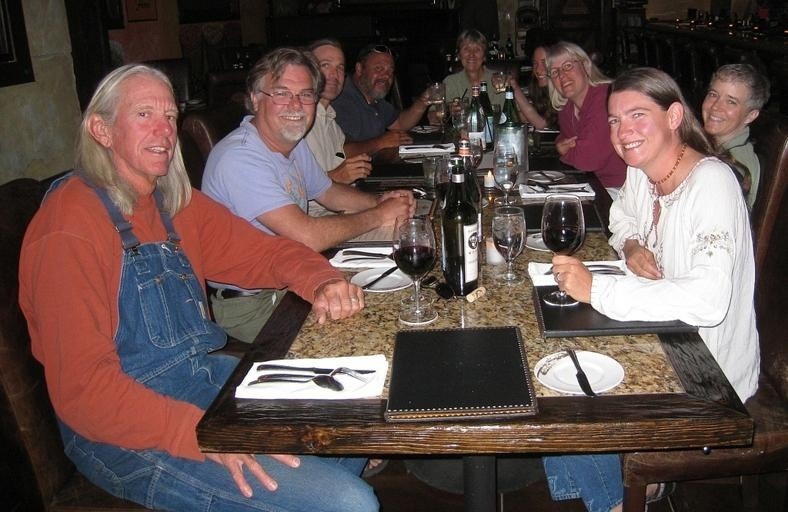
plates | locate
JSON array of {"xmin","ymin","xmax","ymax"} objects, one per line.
[
  {"xmin": 528, "ymin": 169, "xmax": 566, "ymax": 183},
  {"xmin": 523, "ymin": 231, "xmax": 557, "ymax": 254},
  {"xmin": 349, "ymin": 267, "xmax": 418, "ymax": 295},
  {"xmin": 531, "ymin": 348, "xmax": 627, "ymax": 396},
  {"xmin": 411, "ymin": 125, "xmax": 436, "ymax": 134}
]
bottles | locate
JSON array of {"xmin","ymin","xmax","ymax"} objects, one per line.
[
  {"xmin": 440, "ymin": 140, "xmax": 479, "ymax": 295},
  {"xmin": 465, "ymin": 82, "xmax": 521, "ymax": 153}
]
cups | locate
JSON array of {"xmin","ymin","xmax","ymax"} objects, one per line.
[
  {"xmin": 422, "ymin": 153, "xmax": 443, "ymax": 190},
  {"xmin": 449, "ymin": 97, "xmax": 470, "ymax": 130},
  {"xmin": 493, "ymin": 120, "xmax": 530, "ymax": 170},
  {"xmin": 424, "ymin": 71, "xmax": 511, "ymax": 105}
]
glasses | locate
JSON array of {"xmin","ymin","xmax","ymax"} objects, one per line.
[
  {"xmin": 549, "ymin": 60, "xmax": 580, "ymax": 80},
  {"xmin": 259, "ymin": 90, "xmax": 318, "ymax": 105},
  {"xmin": 372, "ymin": 45, "xmax": 392, "ymax": 55}
]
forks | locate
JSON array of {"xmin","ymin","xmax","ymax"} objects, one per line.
[
  {"xmin": 258, "ymin": 367, "xmax": 368, "ymax": 383},
  {"xmin": 405, "ymin": 143, "xmax": 447, "ymax": 150}
]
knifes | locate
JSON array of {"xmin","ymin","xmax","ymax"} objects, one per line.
[
  {"xmin": 257, "ymin": 362, "xmax": 377, "ymax": 374},
  {"xmin": 564, "ymin": 345, "xmax": 596, "ymax": 397},
  {"xmin": 360, "ymin": 267, "xmax": 399, "ymax": 289}
]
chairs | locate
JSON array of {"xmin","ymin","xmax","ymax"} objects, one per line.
[
  {"xmin": 0, "ymin": 166, "xmax": 164, "ymax": 511},
  {"xmin": 180, "ymin": 97, "xmax": 243, "ymax": 154},
  {"xmin": 619, "ymin": 310, "xmax": 786, "ymax": 511},
  {"xmin": 742, "ymin": 118, "xmax": 786, "ymax": 287}
]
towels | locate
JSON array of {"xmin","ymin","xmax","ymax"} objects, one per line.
[
  {"xmin": 328, "ymin": 246, "xmax": 398, "ymax": 270},
  {"xmin": 230, "ymin": 351, "xmax": 389, "ymax": 399},
  {"xmin": 517, "ymin": 182, "xmax": 597, "ymax": 201},
  {"xmin": 526, "ymin": 258, "xmax": 634, "ymax": 288},
  {"xmin": 395, "ymin": 141, "xmax": 458, "ymax": 156}
]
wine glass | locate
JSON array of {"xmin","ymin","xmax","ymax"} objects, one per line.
[
  {"xmin": 493, "ymin": 146, "xmax": 518, "ymax": 204},
  {"xmin": 434, "ymin": 104, "xmax": 451, "ymax": 138},
  {"xmin": 392, "ymin": 211, "xmax": 439, "ymax": 326},
  {"xmin": 490, "ymin": 206, "xmax": 528, "ymax": 286},
  {"xmin": 540, "ymin": 194, "xmax": 587, "ymax": 307}
]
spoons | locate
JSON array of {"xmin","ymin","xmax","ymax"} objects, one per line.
[
  {"xmin": 246, "ymin": 375, "xmax": 344, "ymax": 393},
  {"xmin": 342, "ymin": 249, "xmax": 395, "ymax": 260}
]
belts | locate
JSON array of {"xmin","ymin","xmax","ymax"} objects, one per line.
[{"xmin": 206, "ymin": 285, "xmax": 260, "ymax": 299}]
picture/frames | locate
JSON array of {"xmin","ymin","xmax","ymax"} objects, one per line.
[
  {"xmin": 124, "ymin": 0, "xmax": 159, "ymax": 26},
  {"xmin": 0, "ymin": 0, "xmax": 35, "ymax": 90}
]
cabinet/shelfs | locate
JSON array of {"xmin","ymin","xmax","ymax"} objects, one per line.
[{"xmin": 63, "ymin": 1, "xmax": 242, "ymax": 180}]
